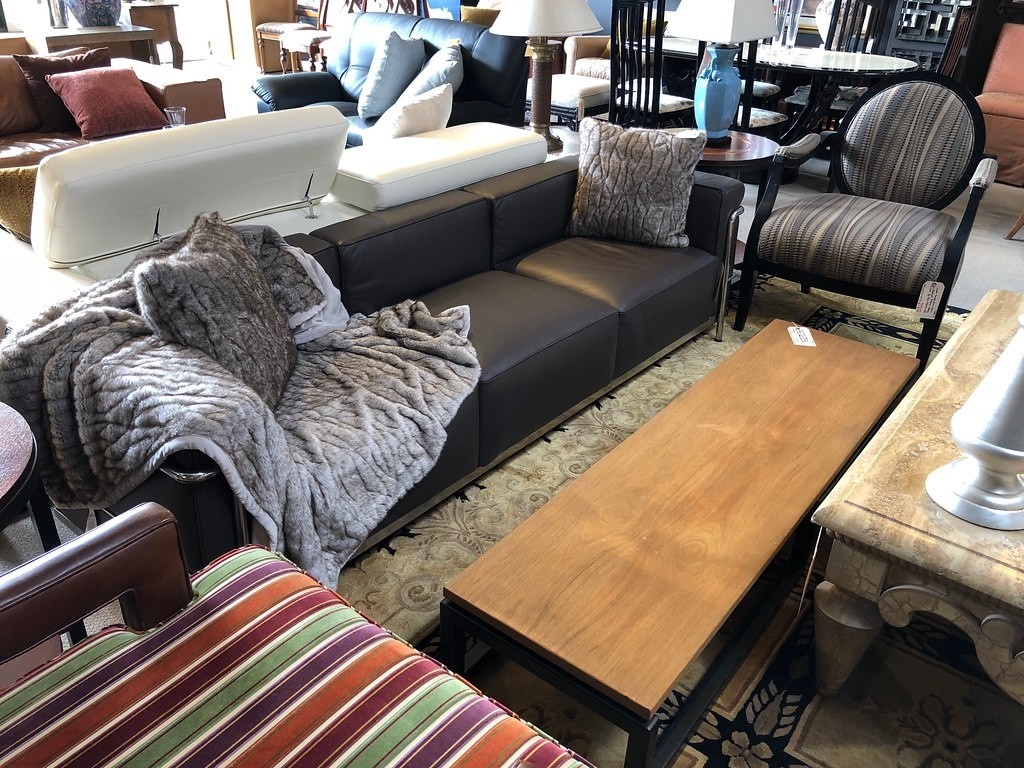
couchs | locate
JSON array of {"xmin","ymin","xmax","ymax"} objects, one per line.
[
  {"xmin": 0, "ymin": 104, "xmax": 545, "ymax": 335},
  {"xmin": 251, "ymin": 16, "xmax": 531, "ymax": 149},
  {"xmin": 56, "ymin": 151, "xmax": 745, "ymax": 551},
  {"xmin": 1, "ymin": 47, "xmax": 226, "ymax": 169}
]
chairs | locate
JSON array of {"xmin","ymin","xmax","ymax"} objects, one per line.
[
  {"xmin": 256, "ymin": 0, "xmax": 414, "ymax": 74},
  {"xmin": 0, "ymin": 499, "xmax": 600, "ymax": 768},
  {"xmin": 608, "ymin": 1, "xmax": 1004, "ymax": 352}
]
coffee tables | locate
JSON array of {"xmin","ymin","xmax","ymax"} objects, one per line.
[{"xmin": 439, "ymin": 319, "xmax": 920, "ymax": 768}]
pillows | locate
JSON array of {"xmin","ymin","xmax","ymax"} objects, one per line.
[
  {"xmin": 12, "ymin": 46, "xmax": 170, "ymax": 140},
  {"xmin": 573, "ymin": 109, "xmax": 706, "ymax": 251},
  {"xmin": 355, "ymin": 30, "xmax": 465, "ymax": 145},
  {"xmin": 132, "ymin": 212, "xmax": 299, "ymax": 419}
]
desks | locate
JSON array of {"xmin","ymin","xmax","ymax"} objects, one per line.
[
  {"xmin": 811, "ymin": 289, "xmax": 1024, "ymax": 698},
  {"xmin": 44, "ymin": 4, "xmax": 185, "ymax": 69},
  {"xmin": 625, "ymin": 35, "xmax": 917, "ymax": 177}
]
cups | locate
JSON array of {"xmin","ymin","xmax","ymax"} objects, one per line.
[
  {"xmin": 164, "ymin": 107, "xmax": 187, "ymax": 124},
  {"xmin": 162, "ymin": 124, "xmax": 185, "ymax": 129}
]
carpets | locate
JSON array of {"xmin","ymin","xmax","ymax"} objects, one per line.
[{"xmin": 327, "ymin": 303, "xmax": 1024, "ymax": 768}]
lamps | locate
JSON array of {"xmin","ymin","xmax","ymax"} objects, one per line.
[
  {"xmin": 665, "ymin": 1, "xmax": 779, "ymax": 148},
  {"xmin": 488, "ymin": 0, "xmax": 604, "ymax": 152}
]
